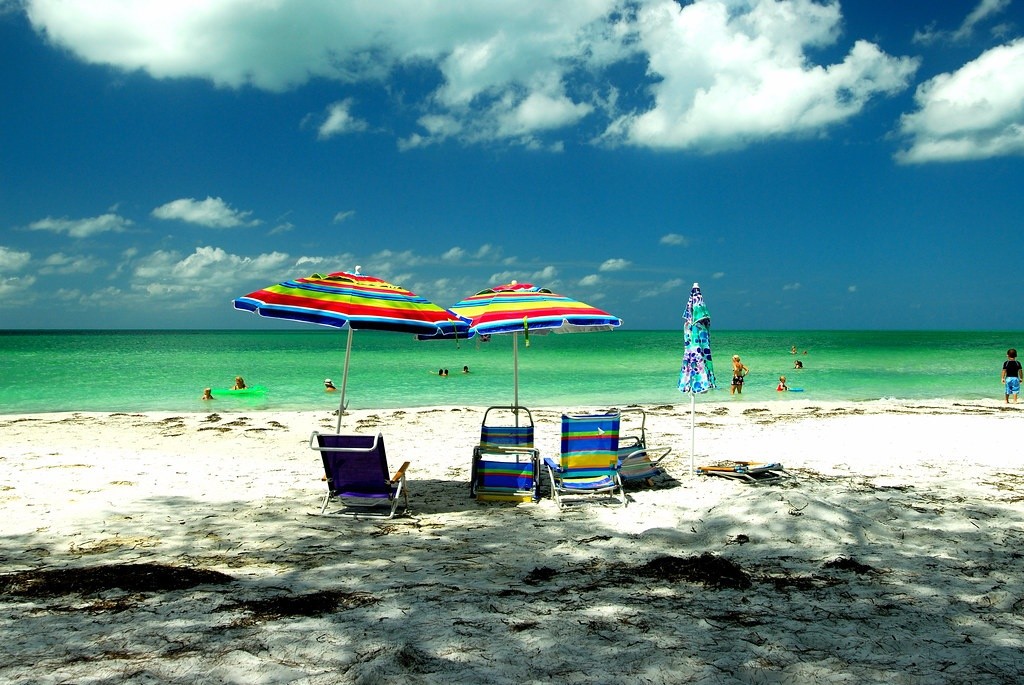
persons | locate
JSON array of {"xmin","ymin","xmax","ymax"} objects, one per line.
[
  {"xmin": 233, "ymin": 377, "xmax": 249, "ymax": 390},
  {"xmin": 792, "ymin": 346, "xmax": 797, "ymax": 354},
  {"xmin": 730, "ymin": 355, "xmax": 749, "ymax": 395},
  {"xmin": 777, "ymin": 376, "xmax": 789, "ymax": 392},
  {"xmin": 438, "ymin": 369, "xmax": 448, "ymax": 376},
  {"xmin": 803, "ymin": 351, "xmax": 808, "ymax": 354},
  {"xmin": 1001, "ymin": 349, "xmax": 1023, "ymax": 403},
  {"xmin": 794, "ymin": 360, "xmax": 803, "ymax": 370},
  {"xmin": 324, "ymin": 377, "xmax": 338, "ymax": 391},
  {"xmin": 480, "ymin": 333, "xmax": 491, "ymax": 342},
  {"xmin": 461, "ymin": 366, "xmax": 469, "ymax": 373},
  {"xmin": 201, "ymin": 388, "xmax": 213, "ymax": 399}
]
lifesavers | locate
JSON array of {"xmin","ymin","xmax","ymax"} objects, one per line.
[
  {"xmin": 787, "ymin": 350, "xmax": 797, "ymax": 354},
  {"xmin": 787, "ymin": 388, "xmax": 804, "ymax": 392}
]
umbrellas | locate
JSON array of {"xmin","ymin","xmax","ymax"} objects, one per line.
[
  {"xmin": 678, "ymin": 283, "xmax": 718, "ymax": 475},
  {"xmin": 415, "ymin": 280, "xmax": 622, "ymax": 462},
  {"xmin": 232, "ymin": 267, "xmax": 470, "ymax": 434}
]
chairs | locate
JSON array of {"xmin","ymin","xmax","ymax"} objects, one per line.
[
  {"xmin": 309, "ymin": 431, "xmax": 411, "ymax": 520},
  {"xmin": 696, "ymin": 459, "xmax": 791, "ymax": 482},
  {"xmin": 470, "ymin": 405, "xmax": 539, "ymax": 504},
  {"xmin": 544, "ymin": 408, "xmax": 672, "ymax": 510}
]
